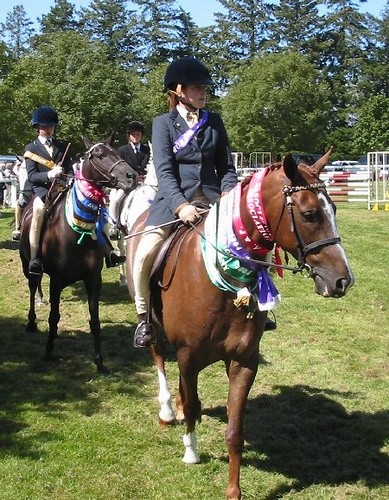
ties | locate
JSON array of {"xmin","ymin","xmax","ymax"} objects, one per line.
[
  {"xmin": 189, "ymin": 114, "xmax": 199, "ymax": 124},
  {"xmin": 47, "ymin": 139, "xmax": 52, "ymax": 148},
  {"xmin": 135, "ymin": 145, "xmax": 139, "ymax": 155}
]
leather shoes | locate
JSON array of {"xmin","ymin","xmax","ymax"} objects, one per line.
[
  {"xmin": 30, "ymin": 262, "xmax": 41, "ymax": 273},
  {"xmin": 134, "ymin": 322, "xmax": 156, "ymax": 346},
  {"xmin": 265, "ymin": 317, "xmax": 275, "ymax": 329},
  {"xmin": 13, "ymin": 230, "xmax": 21, "ymax": 239},
  {"xmin": 105, "ymin": 252, "xmax": 126, "ymax": 269}
]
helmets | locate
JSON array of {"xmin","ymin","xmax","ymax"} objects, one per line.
[
  {"xmin": 126, "ymin": 121, "xmax": 145, "ymax": 133},
  {"xmin": 164, "ymin": 58, "xmax": 215, "ymax": 88},
  {"xmin": 33, "ymin": 107, "xmax": 58, "ymax": 127}
]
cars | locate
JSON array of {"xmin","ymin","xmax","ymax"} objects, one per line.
[
  {"xmin": 0, "ymin": 154, "xmax": 23, "ymax": 167},
  {"xmin": 244, "ymin": 151, "xmax": 389, "ymax": 186}
]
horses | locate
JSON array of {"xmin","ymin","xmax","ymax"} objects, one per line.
[{"xmin": 16, "ymin": 128, "xmax": 356, "ymax": 500}]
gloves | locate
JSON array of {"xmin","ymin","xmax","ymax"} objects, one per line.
[
  {"xmin": 47, "ymin": 162, "xmax": 64, "ymax": 179},
  {"xmin": 178, "ymin": 205, "xmax": 205, "ymax": 225}
]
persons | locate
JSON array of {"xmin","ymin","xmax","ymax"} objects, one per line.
[
  {"xmin": 0, "ymin": 105, "xmax": 151, "ymax": 275},
  {"xmin": 134, "ymin": 58, "xmax": 277, "ymax": 348}
]
